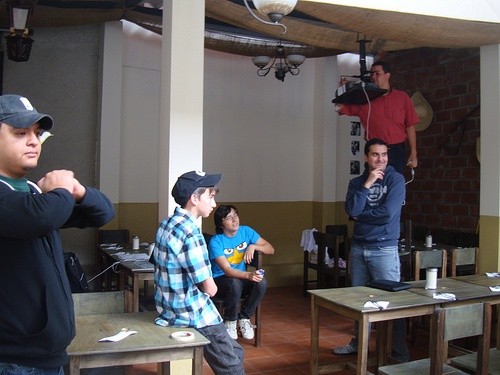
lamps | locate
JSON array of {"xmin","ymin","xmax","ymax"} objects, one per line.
[
  {"xmin": 244, "ymin": 0, "xmax": 298, "ymax": 34},
  {"xmin": 4, "ymin": 0, "xmax": 39, "ymax": 63},
  {"xmin": 253, "ymin": 39, "xmax": 306, "ymax": 82}
]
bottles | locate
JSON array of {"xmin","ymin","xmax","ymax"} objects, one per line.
[
  {"xmin": 132, "ymin": 235, "xmax": 139, "ymax": 250},
  {"xmin": 425, "ymin": 234, "xmax": 432, "ymax": 247},
  {"xmin": 149, "ymin": 242, "xmax": 155, "ymax": 257},
  {"xmin": 426, "ymin": 267, "xmax": 438, "ymax": 289}
]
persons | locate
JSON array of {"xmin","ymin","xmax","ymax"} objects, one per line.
[
  {"xmin": 154, "ymin": 170, "xmax": 245, "ymax": 375},
  {"xmin": 335, "ymin": 58, "xmax": 421, "ymax": 202},
  {"xmin": 333, "ymin": 137, "xmax": 409, "ymax": 363},
  {"xmin": 0, "ymin": 94, "xmax": 116, "ymax": 375},
  {"xmin": 208, "ymin": 204, "xmax": 274, "ymax": 339}
]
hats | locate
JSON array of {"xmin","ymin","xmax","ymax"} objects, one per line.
[
  {"xmin": 171, "ymin": 170, "xmax": 223, "ymax": 208},
  {"xmin": 0, "ymin": 94, "xmax": 54, "ymax": 131}
]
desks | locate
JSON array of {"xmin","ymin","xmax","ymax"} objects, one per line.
[
  {"xmin": 307, "ymin": 273, "xmax": 500, "ymax": 375},
  {"xmin": 66, "ymin": 242, "xmax": 211, "ymax": 375}
]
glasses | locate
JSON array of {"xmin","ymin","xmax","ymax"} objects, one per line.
[{"xmin": 222, "ymin": 213, "xmax": 240, "ymax": 221}]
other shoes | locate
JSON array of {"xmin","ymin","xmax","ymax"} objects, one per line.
[
  {"xmin": 390, "ymin": 356, "xmax": 406, "ymax": 363},
  {"xmin": 333, "ymin": 344, "xmax": 357, "ymax": 355}
]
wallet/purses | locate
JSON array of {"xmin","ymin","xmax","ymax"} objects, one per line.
[{"xmin": 366, "ymin": 278, "xmax": 413, "ymax": 292}]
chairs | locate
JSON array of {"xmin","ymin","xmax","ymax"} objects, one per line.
[
  {"xmin": 97, "ymin": 229, "xmax": 130, "ymax": 292},
  {"xmin": 301, "ymin": 224, "xmax": 351, "ymax": 297},
  {"xmin": 212, "ymin": 251, "xmax": 263, "ymax": 347},
  {"xmin": 408, "ymin": 249, "xmax": 447, "ymax": 337},
  {"xmin": 451, "ymin": 247, "xmax": 479, "ymax": 277},
  {"xmin": 71, "ymin": 291, "xmax": 133, "ymax": 317},
  {"xmin": 377, "ymin": 302, "xmax": 500, "ymax": 375}
]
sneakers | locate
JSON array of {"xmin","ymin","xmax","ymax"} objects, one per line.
[
  {"xmin": 238, "ymin": 319, "xmax": 254, "ymax": 339},
  {"xmin": 224, "ymin": 320, "xmax": 238, "ymax": 340}
]
